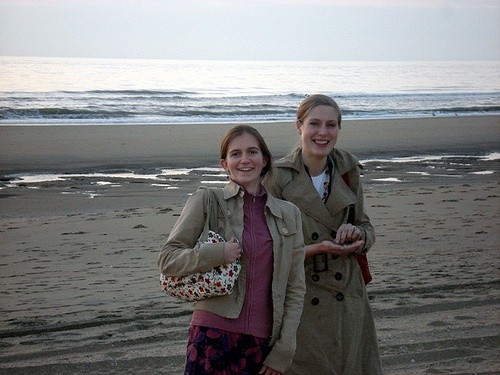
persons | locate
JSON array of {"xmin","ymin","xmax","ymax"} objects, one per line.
[
  {"xmin": 261, "ymin": 94, "xmax": 384, "ymax": 375},
  {"xmin": 157, "ymin": 123, "xmax": 305, "ymax": 375}
]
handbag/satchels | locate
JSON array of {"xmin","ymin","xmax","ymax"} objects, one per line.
[
  {"xmin": 355, "ymin": 251, "xmax": 372, "ymax": 286},
  {"xmin": 159, "ymin": 230, "xmax": 242, "ymax": 302}
]
